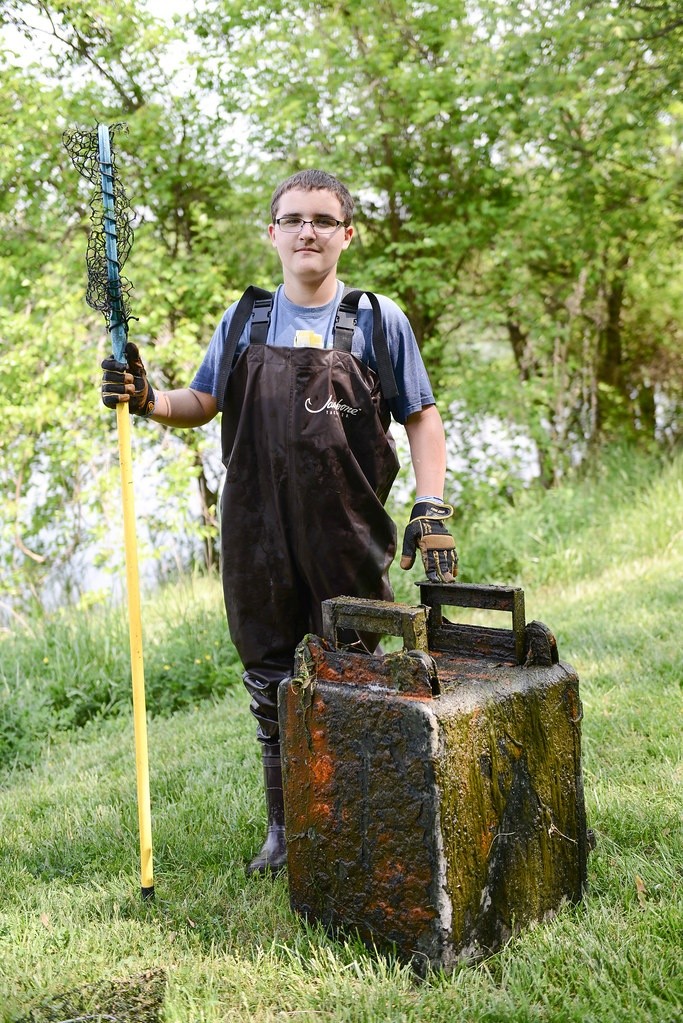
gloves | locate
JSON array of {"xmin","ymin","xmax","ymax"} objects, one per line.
[
  {"xmin": 101, "ymin": 342, "xmax": 158, "ymax": 418},
  {"xmin": 400, "ymin": 497, "xmax": 459, "ymax": 584}
]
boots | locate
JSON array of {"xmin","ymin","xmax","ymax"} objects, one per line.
[{"xmin": 243, "ymin": 745, "xmax": 288, "ymax": 876}]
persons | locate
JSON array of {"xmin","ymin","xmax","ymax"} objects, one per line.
[{"xmin": 102, "ymin": 169, "xmax": 460, "ymax": 879}]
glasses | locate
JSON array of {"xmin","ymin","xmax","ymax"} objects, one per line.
[{"xmin": 272, "ymin": 216, "xmax": 349, "ymax": 235}]
